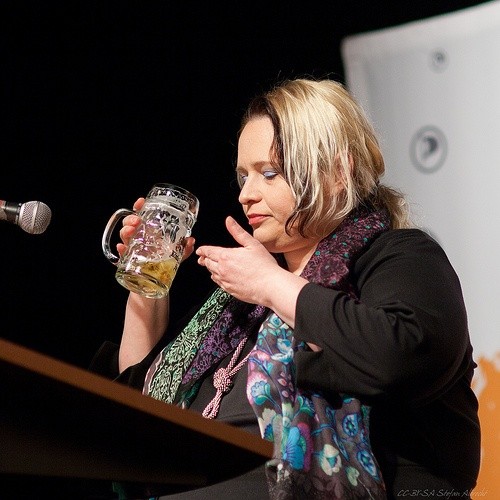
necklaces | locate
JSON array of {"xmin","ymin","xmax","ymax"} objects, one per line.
[{"xmin": 202, "ymin": 303, "xmax": 267, "ymax": 421}]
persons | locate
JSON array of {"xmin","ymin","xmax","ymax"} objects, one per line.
[{"xmin": 114, "ymin": 80, "xmax": 481, "ymax": 500}]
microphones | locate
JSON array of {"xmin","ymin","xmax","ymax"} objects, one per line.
[{"xmin": 1, "ymin": 199, "xmax": 52, "ymax": 234}]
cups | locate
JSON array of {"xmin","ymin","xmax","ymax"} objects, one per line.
[{"xmin": 101, "ymin": 183, "xmax": 199, "ymax": 300}]
